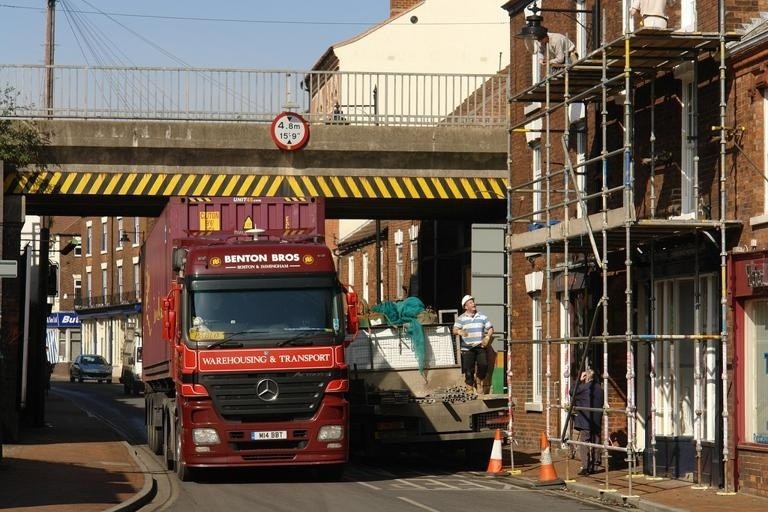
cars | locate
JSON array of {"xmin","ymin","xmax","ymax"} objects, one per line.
[{"xmin": 69, "ymin": 354, "xmax": 112, "ymax": 384}]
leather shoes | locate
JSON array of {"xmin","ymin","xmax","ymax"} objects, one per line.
[{"xmin": 577, "ymin": 468, "xmax": 594, "ymax": 475}]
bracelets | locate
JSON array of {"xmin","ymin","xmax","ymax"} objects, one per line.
[{"xmin": 485, "ymin": 336, "xmax": 490, "ymax": 339}]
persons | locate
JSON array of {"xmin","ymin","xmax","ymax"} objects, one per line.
[
  {"xmin": 452, "ymin": 295, "xmax": 494, "ymax": 394},
  {"xmin": 531, "ymin": 26, "xmax": 577, "ymax": 73},
  {"xmin": 569, "ymin": 369, "xmax": 603, "ymax": 475},
  {"xmin": 629, "ymin": 0, "xmax": 668, "ymax": 29}
]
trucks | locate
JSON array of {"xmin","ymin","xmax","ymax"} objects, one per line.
[
  {"xmin": 142, "ymin": 196, "xmax": 359, "ymax": 482},
  {"xmin": 346, "ymin": 309, "xmax": 514, "ymax": 471},
  {"xmin": 118, "ymin": 326, "xmax": 142, "ymax": 395}
]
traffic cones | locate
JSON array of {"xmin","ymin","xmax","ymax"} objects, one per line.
[
  {"xmin": 532, "ymin": 430, "xmax": 565, "ymax": 486},
  {"xmin": 481, "ymin": 428, "xmax": 510, "ymax": 476}
]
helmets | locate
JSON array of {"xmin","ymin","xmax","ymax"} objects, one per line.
[{"xmin": 462, "ymin": 295, "xmax": 475, "ymax": 307}]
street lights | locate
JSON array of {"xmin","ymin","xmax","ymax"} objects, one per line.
[{"xmin": 120, "ymin": 230, "xmax": 143, "ymax": 242}]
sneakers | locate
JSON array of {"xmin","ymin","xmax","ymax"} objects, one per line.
[{"xmin": 465, "ymin": 378, "xmax": 484, "ymax": 394}]
